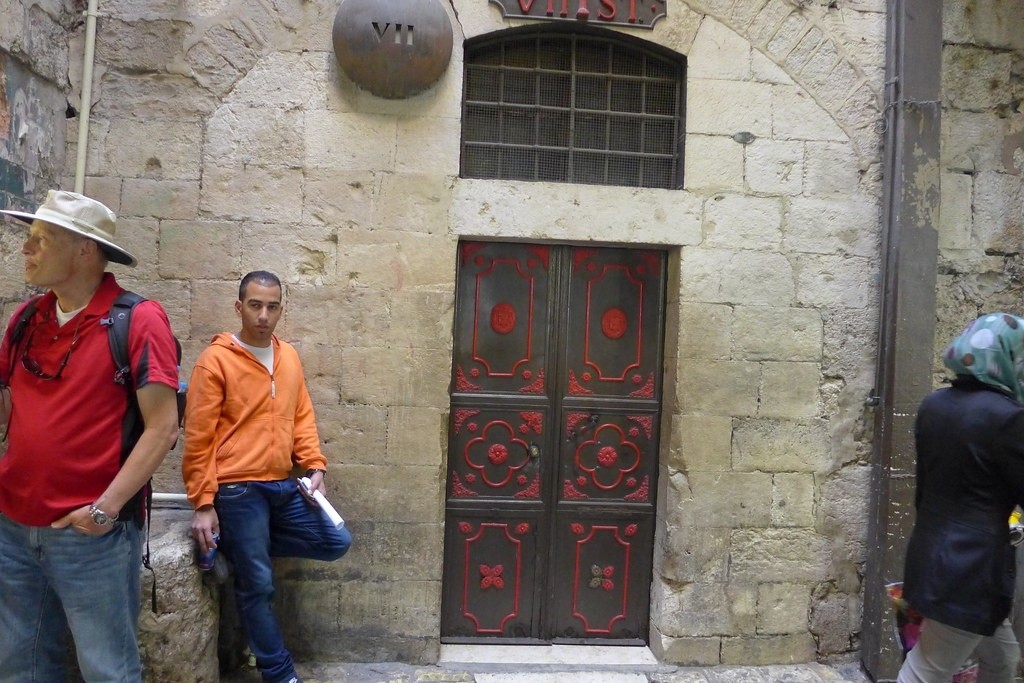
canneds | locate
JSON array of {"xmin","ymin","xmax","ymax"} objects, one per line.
[{"xmin": 198, "ymin": 530, "xmax": 218, "ymax": 570}]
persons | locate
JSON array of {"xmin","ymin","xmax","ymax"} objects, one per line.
[
  {"xmin": 895, "ymin": 311, "xmax": 1024, "ymax": 683},
  {"xmin": 0, "ymin": 189, "xmax": 181, "ymax": 683},
  {"xmin": 181, "ymin": 271, "xmax": 349, "ymax": 683}
]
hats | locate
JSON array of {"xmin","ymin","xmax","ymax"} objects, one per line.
[{"xmin": 1, "ymin": 189, "xmax": 139, "ymax": 269}]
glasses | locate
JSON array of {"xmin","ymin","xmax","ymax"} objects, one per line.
[{"xmin": 20, "ymin": 320, "xmax": 82, "ymax": 379}]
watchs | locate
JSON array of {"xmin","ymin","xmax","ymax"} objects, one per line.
[{"xmin": 89, "ymin": 506, "xmax": 119, "ymax": 526}]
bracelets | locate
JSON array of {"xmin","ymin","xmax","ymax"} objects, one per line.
[{"xmin": 306, "ymin": 469, "xmax": 325, "ymax": 477}]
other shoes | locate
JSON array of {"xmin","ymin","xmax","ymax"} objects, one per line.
[{"xmin": 214, "ymin": 548, "xmax": 234, "ymax": 585}]
